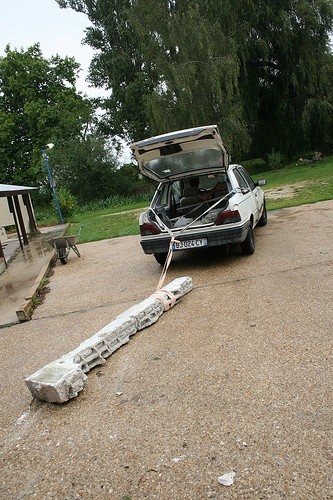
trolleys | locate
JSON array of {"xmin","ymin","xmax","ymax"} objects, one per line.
[{"xmin": 46, "ymin": 225, "xmax": 82, "ymax": 265}]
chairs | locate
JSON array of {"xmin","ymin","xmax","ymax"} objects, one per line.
[{"xmin": 180, "ymin": 182, "xmax": 228, "ymax": 218}]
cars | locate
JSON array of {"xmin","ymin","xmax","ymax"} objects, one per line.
[{"xmin": 129, "ymin": 125, "xmax": 268, "ymax": 266}]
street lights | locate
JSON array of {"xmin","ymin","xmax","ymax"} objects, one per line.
[{"xmin": 42, "ymin": 141, "xmax": 63, "ymax": 222}]
